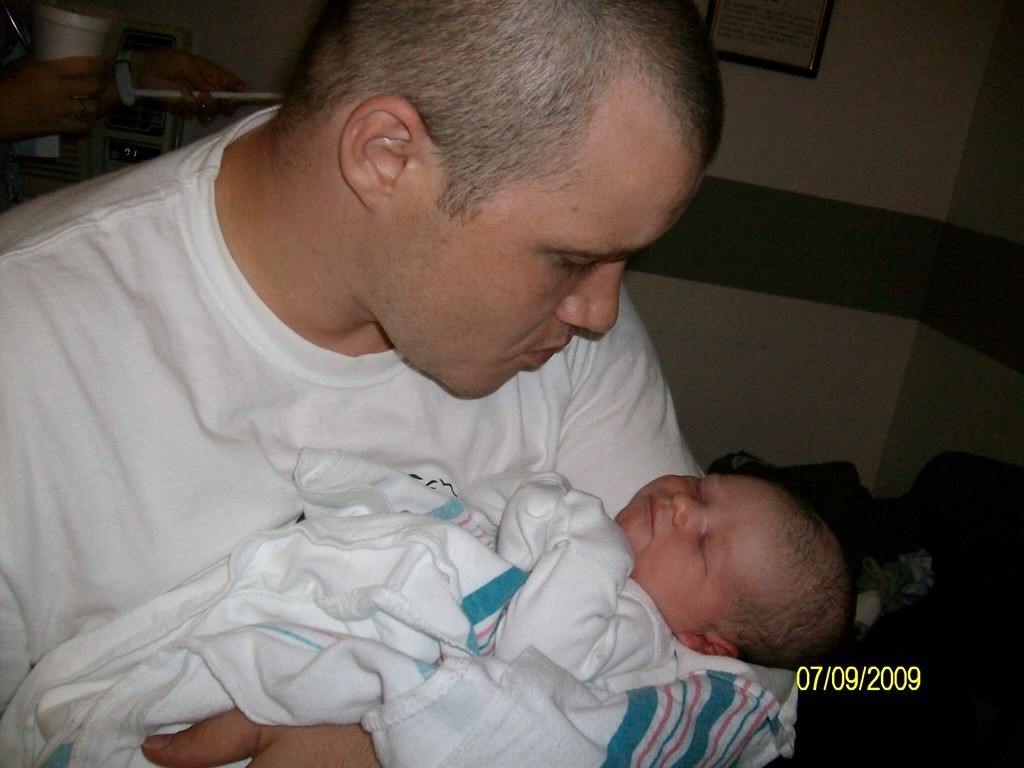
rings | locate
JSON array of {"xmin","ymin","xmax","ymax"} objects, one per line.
[
  {"xmin": 199, "ymin": 113, "xmax": 213, "ymax": 122},
  {"xmin": 74, "ymin": 96, "xmax": 85, "ymax": 120}
]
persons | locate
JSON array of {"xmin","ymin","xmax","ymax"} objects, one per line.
[
  {"xmin": 0, "ymin": 0, "xmax": 799, "ymax": 768},
  {"xmin": 0, "ymin": 472, "xmax": 848, "ymax": 768}
]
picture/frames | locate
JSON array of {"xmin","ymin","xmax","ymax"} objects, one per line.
[{"xmin": 706, "ymin": 0, "xmax": 835, "ymax": 80}]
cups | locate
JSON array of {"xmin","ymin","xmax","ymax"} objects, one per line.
[{"xmin": 27, "ymin": 2, "xmax": 116, "ymax": 104}]
